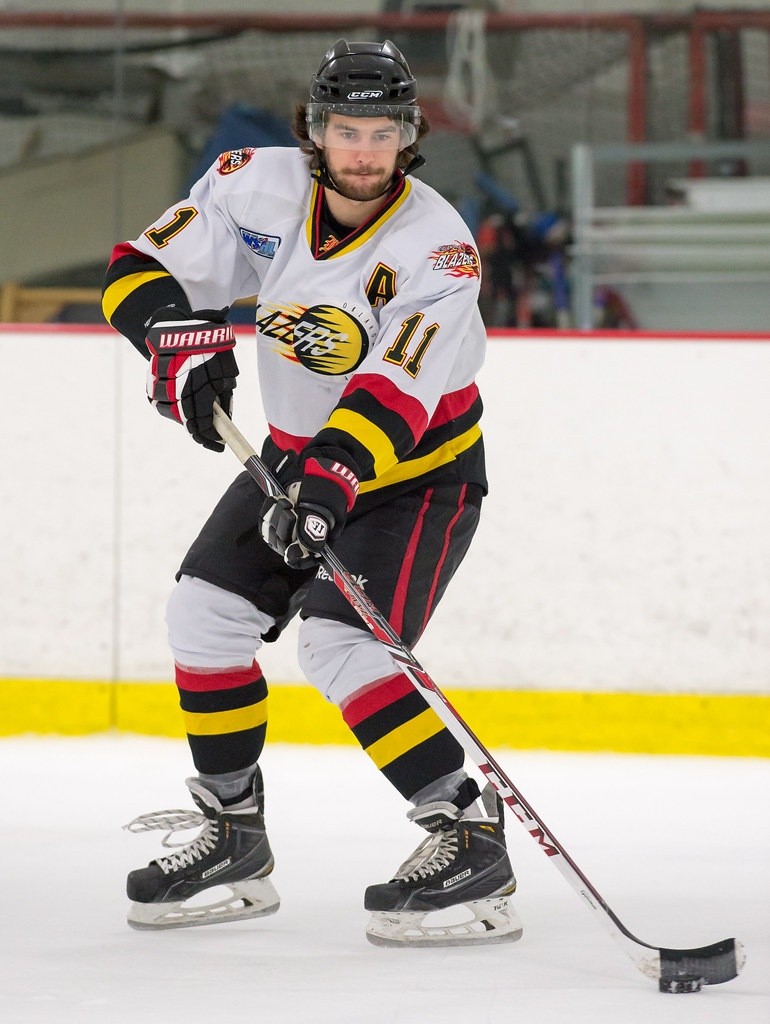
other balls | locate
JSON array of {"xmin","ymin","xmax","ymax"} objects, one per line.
[{"xmin": 657, "ymin": 974, "xmax": 705, "ymax": 995}]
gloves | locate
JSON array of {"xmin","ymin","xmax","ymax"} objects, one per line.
[
  {"xmin": 259, "ymin": 448, "xmax": 362, "ymax": 569},
  {"xmin": 143, "ymin": 305, "xmax": 240, "ymax": 453}
]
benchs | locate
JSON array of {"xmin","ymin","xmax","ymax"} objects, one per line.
[{"xmin": 572, "ymin": 139, "xmax": 770, "ymax": 332}]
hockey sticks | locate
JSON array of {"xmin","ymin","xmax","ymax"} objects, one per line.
[{"xmin": 209, "ymin": 399, "xmax": 750, "ymax": 990}]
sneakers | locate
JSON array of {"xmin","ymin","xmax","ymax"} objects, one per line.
[
  {"xmin": 121, "ymin": 763, "xmax": 280, "ymax": 933},
  {"xmin": 363, "ymin": 780, "xmax": 523, "ymax": 949}
]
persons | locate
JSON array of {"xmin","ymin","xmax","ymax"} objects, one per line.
[{"xmin": 99, "ymin": 40, "xmax": 524, "ymax": 947}]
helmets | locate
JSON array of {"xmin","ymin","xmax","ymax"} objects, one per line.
[{"xmin": 304, "ymin": 38, "xmax": 421, "ymax": 139}]
